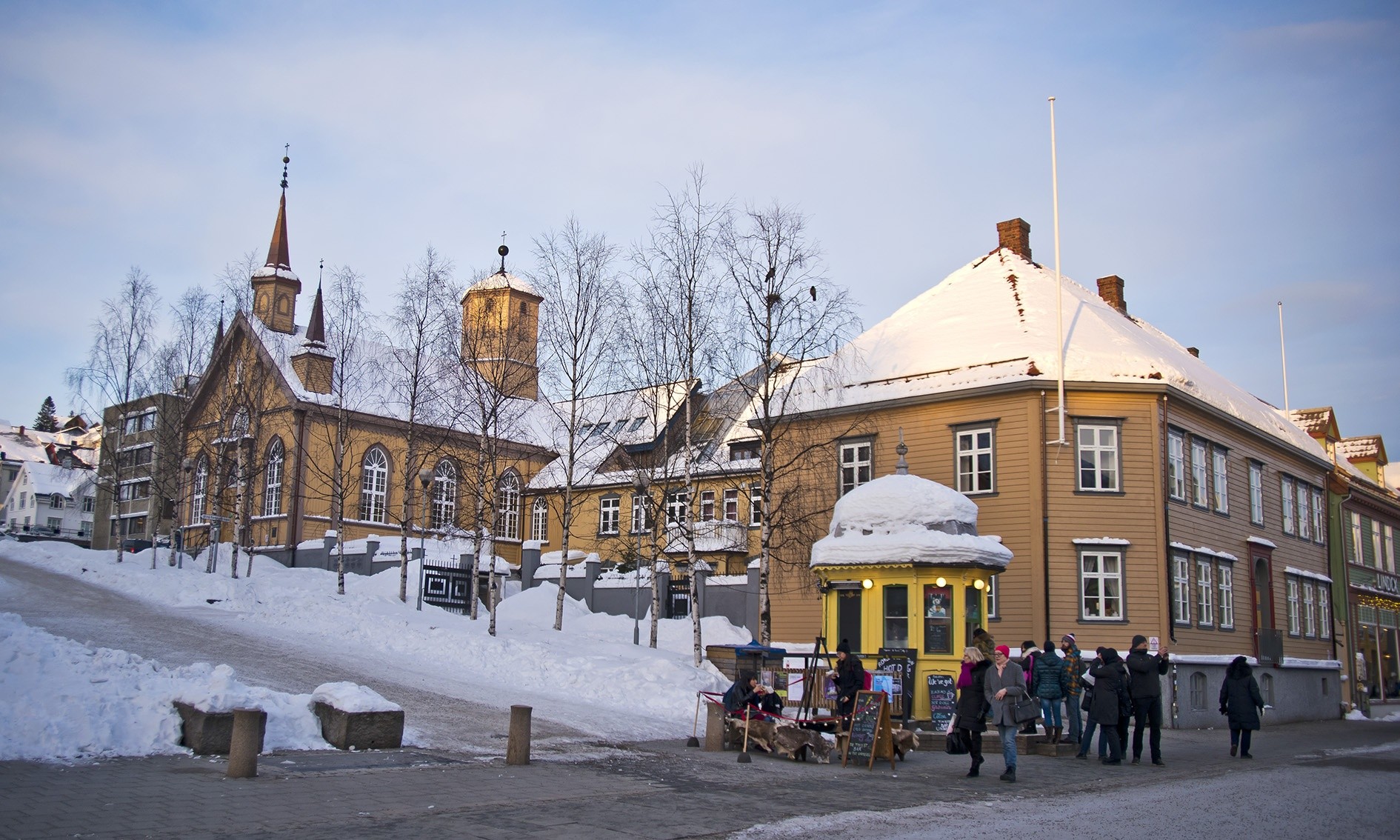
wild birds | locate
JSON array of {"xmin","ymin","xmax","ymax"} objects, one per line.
[
  {"xmin": 810, "ymin": 286, "xmax": 817, "ymax": 301},
  {"xmin": 765, "ymin": 267, "xmax": 776, "ymax": 283}
]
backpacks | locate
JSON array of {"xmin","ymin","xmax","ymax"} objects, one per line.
[
  {"xmin": 852, "ymin": 661, "xmax": 872, "ymax": 691},
  {"xmin": 762, "ymin": 692, "xmax": 784, "ymax": 717}
]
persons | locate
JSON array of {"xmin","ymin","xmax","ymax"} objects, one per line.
[
  {"xmin": 1017, "ymin": 640, "xmax": 1042, "ymax": 735},
  {"xmin": 1059, "ymin": 633, "xmax": 1085, "ymax": 745},
  {"xmin": 984, "ymin": 645, "xmax": 1027, "ymax": 781},
  {"xmin": 1032, "ymin": 640, "xmax": 1064, "ymax": 746},
  {"xmin": 830, "ymin": 639, "xmax": 863, "ymax": 761},
  {"xmin": 1075, "ymin": 646, "xmax": 1131, "ymax": 765},
  {"xmin": 1219, "ymin": 656, "xmax": 1265, "ymax": 758},
  {"xmin": 956, "ymin": 647, "xmax": 993, "ymax": 778},
  {"xmin": 725, "ymin": 669, "xmax": 776, "ymax": 754},
  {"xmin": 972, "ymin": 627, "xmax": 996, "ymax": 722},
  {"xmin": 1126, "ymin": 635, "xmax": 1170, "ymax": 767}
]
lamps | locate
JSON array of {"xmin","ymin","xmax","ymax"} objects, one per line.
[
  {"xmin": 986, "ymin": 585, "xmax": 991, "ymax": 592},
  {"xmin": 936, "ymin": 577, "xmax": 946, "ymax": 587},
  {"xmin": 972, "ymin": 579, "xmax": 984, "ymax": 590},
  {"xmin": 863, "ymin": 579, "xmax": 873, "ymax": 589}
]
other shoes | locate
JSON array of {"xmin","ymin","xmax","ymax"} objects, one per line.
[
  {"xmin": 754, "ymin": 744, "xmax": 775, "ymax": 753},
  {"xmin": 838, "ymin": 754, "xmax": 851, "ymax": 760},
  {"xmin": 967, "ymin": 724, "xmax": 1166, "ymax": 783},
  {"xmin": 1241, "ymin": 753, "xmax": 1253, "ymax": 759},
  {"xmin": 1230, "ymin": 745, "xmax": 1237, "ymax": 756}
]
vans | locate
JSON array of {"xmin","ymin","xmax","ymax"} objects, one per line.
[{"xmin": 121, "ymin": 537, "xmax": 161, "ymax": 554}]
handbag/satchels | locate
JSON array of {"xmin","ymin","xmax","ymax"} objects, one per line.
[
  {"xmin": 825, "ymin": 676, "xmax": 838, "ymax": 700},
  {"xmin": 1081, "ymin": 664, "xmax": 1103, "ymax": 686},
  {"xmin": 943, "ymin": 725, "xmax": 969, "ymax": 754},
  {"xmin": 1009, "ymin": 690, "xmax": 1042, "ymax": 724},
  {"xmin": 1023, "ymin": 655, "xmax": 1033, "ymax": 682}
]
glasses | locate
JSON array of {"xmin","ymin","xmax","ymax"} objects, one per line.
[
  {"xmin": 836, "ymin": 653, "xmax": 842, "ymax": 657},
  {"xmin": 964, "ymin": 654, "xmax": 968, "ymax": 656},
  {"xmin": 994, "ymin": 653, "xmax": 1003, "ymax": 657}
]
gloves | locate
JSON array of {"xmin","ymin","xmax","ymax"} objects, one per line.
[
  {"xmin": 1220, "ymin": 703, "xmax": 1227, "ymax": 715},
  {"xmin": 1259, "ymin": 705, "xmax": 1265, "ymax": 717}
]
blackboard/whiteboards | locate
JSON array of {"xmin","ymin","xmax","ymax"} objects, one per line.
[
  {"xmin": 927, "ymin": 675, "xmax": 957, "ymax": 732},
  {"xmin": 877, "ymin": 647, "xmax": 918, "ymax": 720},
  {"xmin": 845, "ymin": 690, "xmax": 893, "ymax": 759}
]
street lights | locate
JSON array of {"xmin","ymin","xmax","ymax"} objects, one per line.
[
  {"xmin": 631, "ymin": 472, "xmax": 649, "ymax": 645},
  {"xmin": 416, "ymin": 469, "xmax": 435, "ymax": 611},
  {"xmin": 178, "ymin": 457, "xmax": 196, "ymax": 570}
]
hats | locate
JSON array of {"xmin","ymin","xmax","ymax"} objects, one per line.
[
  {"xmin": 1044, "ymin": 641, "xmax": 1055, "ymax": 651},
  {"xmin": 836, "ymin": 639, "xmax": 851, "ymax": 653},
  {"xmin": 995, "ymin": 645, "xmax": 1010, "ymax": 659},
  {"xmin": 1062, "ymin": 632, "xmax": 1076, "ymax": 646},
  {"xmin": 1132, "ymin": 635, "xmax": 1147, "ymax": 647},
  {"xmin": 1100, "ymin": 648, "xmax": 1117, "ymax": 662}
]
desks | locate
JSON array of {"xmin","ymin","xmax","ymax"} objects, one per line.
[{"xmin": 794, "ymin": 721, "xmax": 837, "ymax": 762}]
